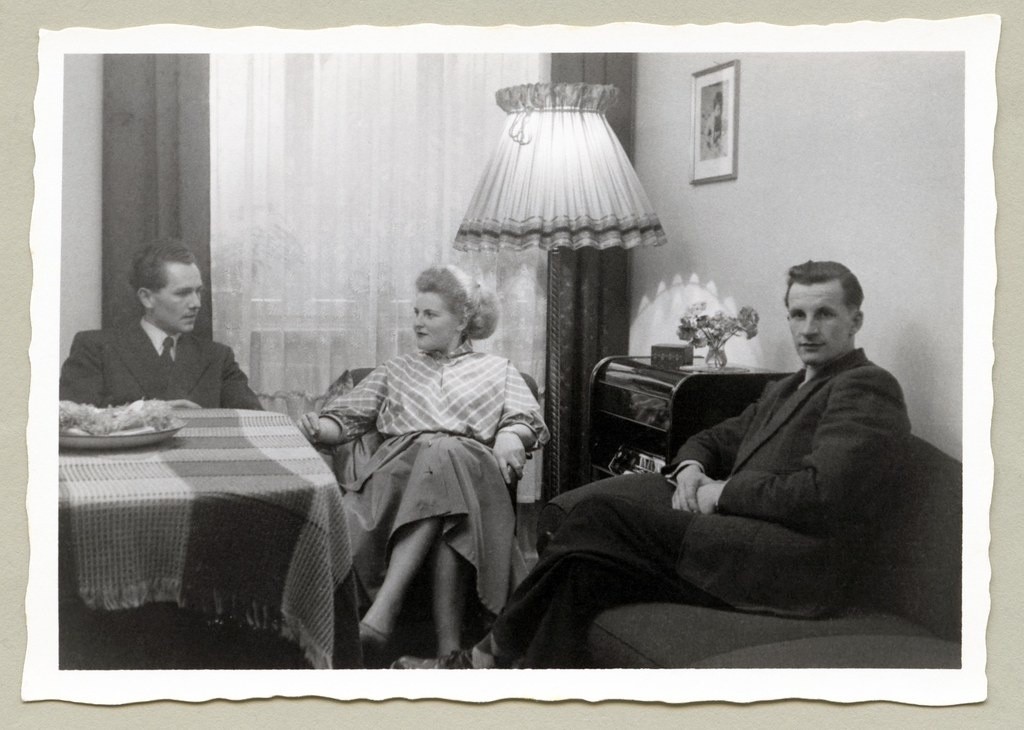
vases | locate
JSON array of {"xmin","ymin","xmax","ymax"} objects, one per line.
[{"xmin": 703, "ymin": 342, "xmax": 730, "ymax": 369}]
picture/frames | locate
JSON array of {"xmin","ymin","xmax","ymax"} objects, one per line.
[{"xmin": 688, "ymin": 59, "xmax": 742, "ymax": 187}]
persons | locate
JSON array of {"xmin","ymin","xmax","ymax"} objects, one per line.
[
  {"xmin": 293, "ymin": 265, "xmax": 551, "ymax": 662},
  {"xmin": 389, "ymin": 260, "xmax": 911, "ymax": 669},
  {"xmin": 60, "ymin": 235, "xmax": 266, "ymax": 410}
]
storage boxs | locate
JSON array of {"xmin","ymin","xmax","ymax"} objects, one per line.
[{"xmin": 649, "ymin": 344, "xmax": 695, "ymax": 370}]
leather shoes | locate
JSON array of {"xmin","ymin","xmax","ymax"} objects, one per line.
[{"xmin": 389, "ymin": 646, "xmax": 474, "ymax": 670}]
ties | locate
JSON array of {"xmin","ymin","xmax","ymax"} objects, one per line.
[{"xmin": 160, "ymin": 337, "xmax": 177, "ymax": 378}]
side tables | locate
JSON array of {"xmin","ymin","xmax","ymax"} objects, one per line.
[{"xmin": 585, "ymin": 352, "xmax": 800, "ymax": 479}]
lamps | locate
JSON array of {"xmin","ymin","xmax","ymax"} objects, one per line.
[{"xmin": 452, "ymin": 78, "xmax": 669, "ymax": 495}]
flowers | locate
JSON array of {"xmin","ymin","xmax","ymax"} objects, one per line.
[{"xmin": 675, "ymin": 302, "xmax": 764, "ymax": 367}]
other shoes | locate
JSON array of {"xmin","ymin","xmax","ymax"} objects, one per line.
[{"xmin": 359, "ymin": 621, "xmax": 392, "ymax": 651}]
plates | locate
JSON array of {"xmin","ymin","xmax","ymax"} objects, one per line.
[{"xmin": 60, "ymin": 417, "xmax": 186, "ymax": 447}]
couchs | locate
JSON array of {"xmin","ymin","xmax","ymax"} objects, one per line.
[{"xmin": 581, "ymin": 424, "xmax": 963, "ymax": 669}]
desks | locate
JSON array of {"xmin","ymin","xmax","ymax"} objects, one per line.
[{"xmin": 59, "ymin": 404, "xmax": 365, "ymax": 670}]
